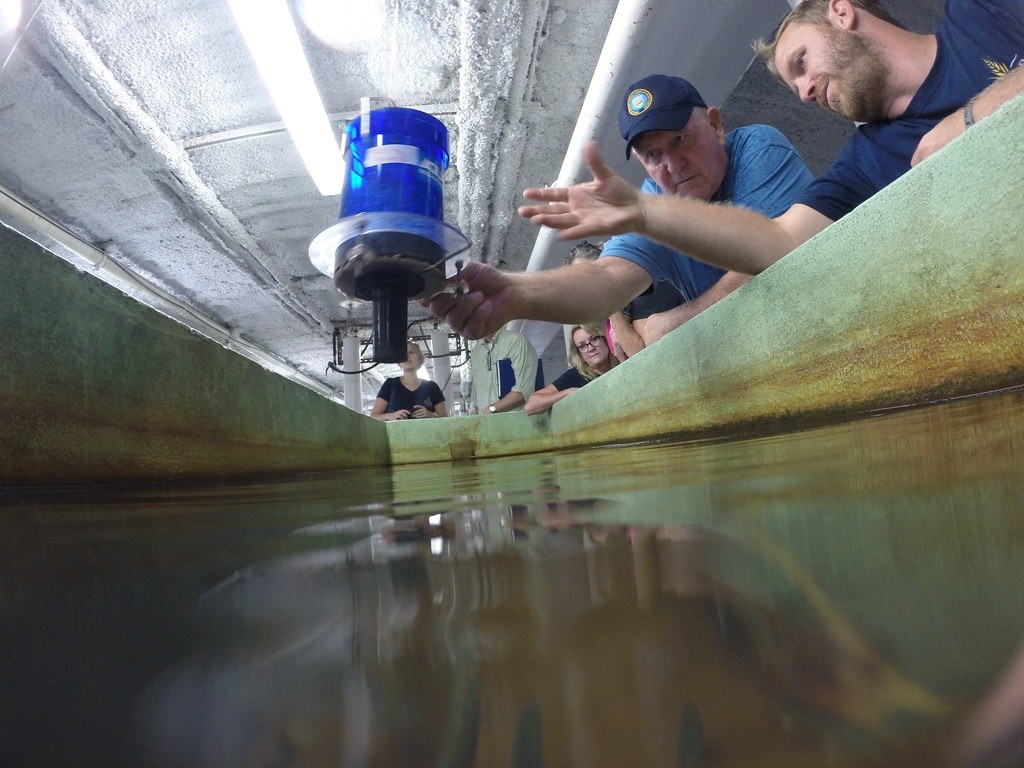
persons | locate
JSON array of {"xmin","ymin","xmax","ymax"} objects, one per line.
[
  {"xmin": 369, "ymin": 340, "xmax": 448, "ymax": 424},
  {"xmin": 418, "ymin": 0, "xmax": 813, "ymax": 359},
  {"xmin": 518, "ymin": 0, "xmax": 1024, "ymax": 276},
  {"xmin": 525, "ymin": 322, "xmax": 619, "ymax": 416},
  {"xmin": 470, "ymin": 325, "xmax": 539, "ymax": 417}
]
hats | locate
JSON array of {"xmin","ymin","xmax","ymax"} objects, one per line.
[{"xmin": 619, "ymin": 74, "xmax": 708, "ymax": 160}]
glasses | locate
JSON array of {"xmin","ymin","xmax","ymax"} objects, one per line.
[{"xmin": 576, "ymin": 335, "xmax": 605, "ymax": 353}]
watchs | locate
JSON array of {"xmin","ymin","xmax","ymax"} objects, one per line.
[{"xmin": 489, "ymin": 404, "xmax": 497, "ymax": 415}]
